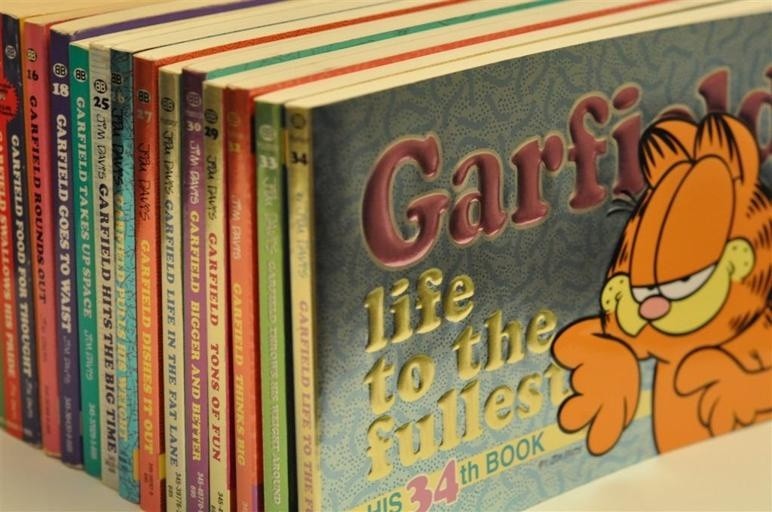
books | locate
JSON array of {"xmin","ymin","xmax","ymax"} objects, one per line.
[{"xmin": 0, "ymin": 2, "xmax": 772, "ymax": 512}]
